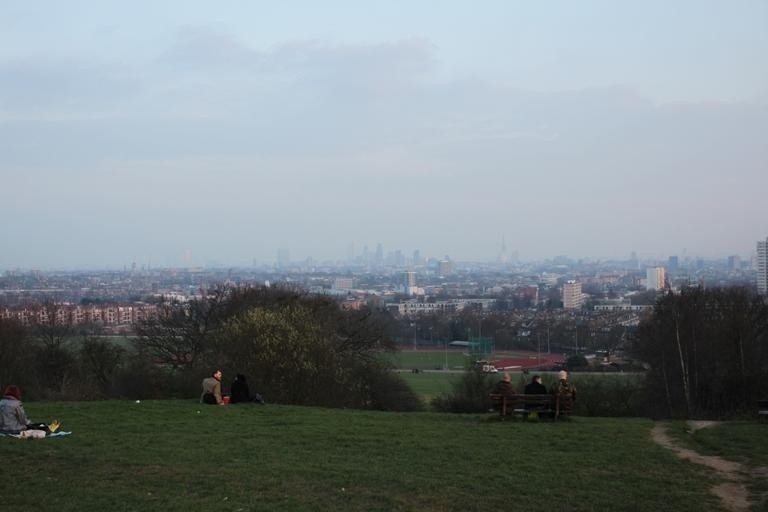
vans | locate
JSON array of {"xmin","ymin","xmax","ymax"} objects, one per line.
[{"xmin": 483, "ymin": 365, "xmax": 498, "ymax": 373}]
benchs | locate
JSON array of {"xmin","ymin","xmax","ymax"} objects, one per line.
[{"xmin": 488, "ymin": 394, "xmax": 573, "ymax": 421}]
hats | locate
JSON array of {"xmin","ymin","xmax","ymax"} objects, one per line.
[{"xmin": 558, "ymin": 370, "xmax": 569, "ymax": 380}]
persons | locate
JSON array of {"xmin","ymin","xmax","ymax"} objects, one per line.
[
  {"xmin": 548, "ymin": 369, "xmax": 578, "ymax": 417},
  {"xmin": 199, "ymin": 367, "xmax": 225, "ymax": 406},
  {"xmin": 521, "ymin": 374, "xmax": 547, "ymax": 423},
  {"xmin": 227, "ymin": 372, "xmax": 250, "ymax": 403},
  {"xmin": 488, "ymin": 372, "xmax": 518, "ymax": 416},
  {"xmin": 0, "ymin": 383, "xmax": 28, "ymax": 434}
]
